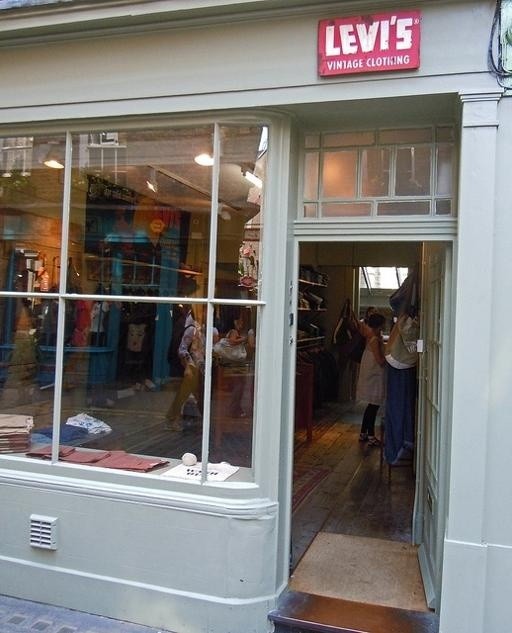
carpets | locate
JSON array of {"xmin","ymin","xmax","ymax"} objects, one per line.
[
  {"xmin": 291, "ymin": 458, "xmax": 333, "ymax": 514},
  {"xmin": 288, "ymin": 531, "xmax": 431, "ymax": 612}
]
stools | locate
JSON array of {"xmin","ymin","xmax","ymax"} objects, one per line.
[{"xmin": 379, "ymin": 416, "xmax": 416, "ymax": 481}]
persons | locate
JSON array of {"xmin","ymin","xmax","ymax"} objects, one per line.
[
  {"xmin": 380, "ymin": 297, "xmax": 421, "ymax": 466},
  {"xmin": 355, "ymin": 312, "xmax": 387, "ymax": 448},
  {"xmin": 353, "ymin": 305, "xmax": 378, "ymax": 365},
  {"xmin": 219, "ymin": 313, "xmax": 248, "ymax": 419},
  {"xmin": 165, "ymin": 303, "xmax": 212, "ymax": 423}
]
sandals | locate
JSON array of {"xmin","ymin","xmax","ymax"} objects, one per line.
[{"xmin": 359, "ymin": 433, "xmax": 381, "ymax": 446}]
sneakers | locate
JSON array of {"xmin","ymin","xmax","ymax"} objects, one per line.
[{"xmin": 163, "ymin": 422, "xmax": 183, "ymax": 431}]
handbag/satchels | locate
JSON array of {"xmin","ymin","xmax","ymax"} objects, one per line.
[
  {"xmin": 214, "ymin": 338, "xmax": 247, "ymax": 362},
  {"xmin": 332, "ymin": 316, "xmax": 361, "ymax": 343},
  {"xmin": 350, "ymin": 341, "xmax": 364, "ymax": 363},
  {"xmin": 384, "ymin": 313, "xmax": 420, "ymax": 369},
  {"xmin": 390, "ymin": 285, "xmax": 410, "ymax": 311},
  {"xmin": 175, "ymin": 323, "xmax": 195, "ymax": 353}
]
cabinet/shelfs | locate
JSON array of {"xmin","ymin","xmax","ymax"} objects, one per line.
[{"xmin": 297, "ymin": 279, "xmax": 327, "ymax": 343}]
